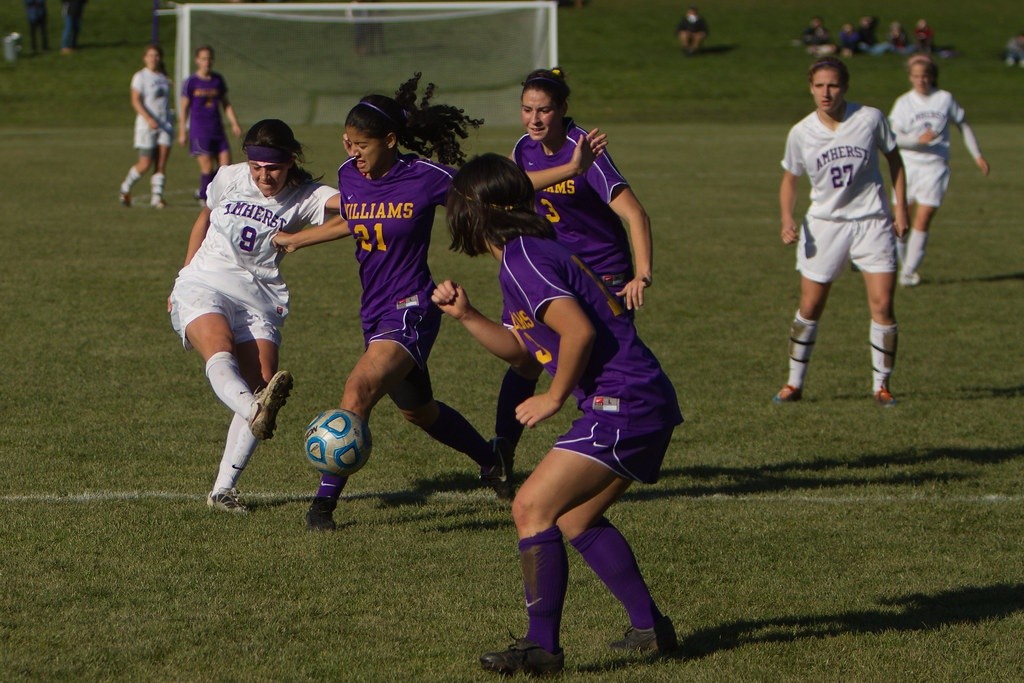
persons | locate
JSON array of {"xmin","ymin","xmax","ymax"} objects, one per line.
[
  {"xmin": 168, "ymin": 118, "xmax": 353, "ymax": 517},
  {"xmin": 482, "ymin": 64, "xmax": 655, "ymax": 475},
  {"xmin": 25, "ymin": 0, "xmax": 51, "ymax": 56},
  {"xmin": 118, "ymin": 45, "xmax": 173, "ymax": 209},
  {"xmin": 177, "ymin": 48, "xmax": 241, "ymax": 204},
  {"xmin": 773, "ymin": 58, "xmax": 911, "ymax": 409},
  {"xmin": 884, "ymin": 54, "xmax": 990, "ymax": 286},
  {"xmin": 1004, "ymin": 33, "xmax": 1024, "ymax": 68},
  {"xmin": 676, "ymin": 8, "xmax": 709, "ymax": 56},
  {"xmin": 273, "ymin": 74, "xmax": 516, "ymax": 532},
  {"xmin": 60, "ymin": 0, "xmax": 89, "ymax": 53},
  {"xmin": 431, "ymin": 152, "xmax": 685, "ymax": 680},
  {"xmin": 802, "ymin": 14, "xmax": 936, "ymax": 56}
]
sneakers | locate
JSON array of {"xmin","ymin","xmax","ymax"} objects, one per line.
[
  {"xmin": 481, "ymin": 628, "xmax": 564, "ymax": 675},
  {"xmin": 873, "ymin": 386, "xmax": 895, "ymax": 405},
  {"xmin": 248, "ymin": 370, "xmax": 294, "ymax": 440},
  {"xmin": 479, "ymin": 437, "xmax": 512, "ymax": 498},
  {"xmin": 207, "ymin": 488, "xmax": 250, "ymax": 514},
  {"xmin": 307, "ymin": 495, "xmax": 338, "ymax": 530},
  {"xmin": 773, "ymin": 385, "xmax": 802, "ymax": 403},
  {"xmin": 610, "ymin": 616, "xmax": 677, "ymax": 654}
]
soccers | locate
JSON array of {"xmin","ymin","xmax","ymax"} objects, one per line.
[{"xmin": 302, "ymin": 408, "xmax": 373, "ymax": 476}]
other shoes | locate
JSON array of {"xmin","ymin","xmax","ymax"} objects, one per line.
[
  {"xmin": 193, "ymin": 188, "xmax": 204, "ymax": 204},
  {"xmin": 898, "ymin": 272, "xmax": 921, "ymax": 286},
  {"xmin": 119, "ymin": 183, "xmax": 133, "ymax": 207},
  {"xmin": 59, "ymin": 47, "xmax": 73, "ymax": 57},
  {"xmin": 150, "ymin": 195, "xmax": 168, "ymax": 209}
]
